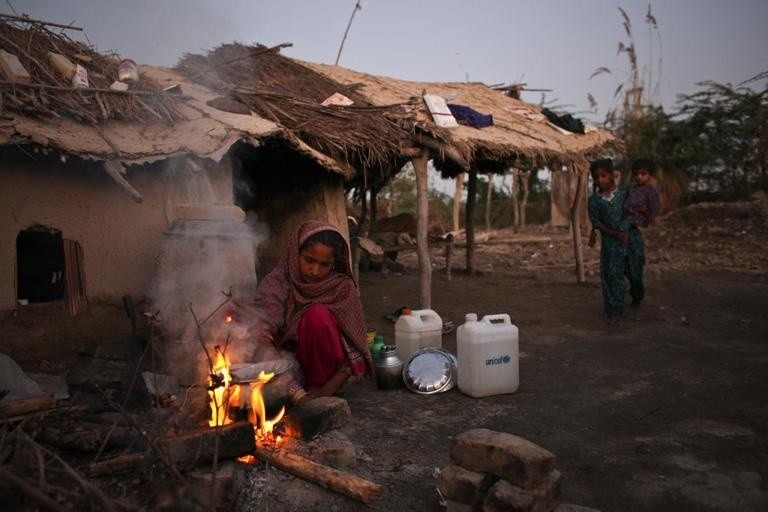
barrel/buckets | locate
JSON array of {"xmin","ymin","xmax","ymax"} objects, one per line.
[
  {"xmin": 395, "ymin": 307, "xmax": 442, "ymax": 366},
  {"xmin": 457, "ymin": 313, "xmax": 520, "ymax": 397}
]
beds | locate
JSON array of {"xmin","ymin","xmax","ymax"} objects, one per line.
[{"xmin": 367, "ymin": 214, "xmax": 454, "ymax": 282}]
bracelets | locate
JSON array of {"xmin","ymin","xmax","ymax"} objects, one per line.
[{"xmin": 285, "ymin": 379, "xmax": 311, "ymax": 404}]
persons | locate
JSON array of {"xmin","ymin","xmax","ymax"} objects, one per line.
[
  {"xmin": 255, "ymin": 217, "xmax": 378, "ymax": 405},
  {"xmin": 623, "ymin": 158, "xmax": 662, "ymax": 224},
  {"xmin": 588, "ymin": 156, "xmax": 647, "ymax": 321}
]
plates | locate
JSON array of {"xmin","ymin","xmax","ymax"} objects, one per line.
[
  {"xmin": 236, "ymin": 358, "xmax": 295, "ymax": 383},
  {"xmin": 402, "ymin": 349, "xmax": 451, "ymax": 395},
  {"xmin": 440, "ymin": 348, "xmax": 457, "ymax": 393}
]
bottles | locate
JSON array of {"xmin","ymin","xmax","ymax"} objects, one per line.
[
  {"xmin": 371, "ymin": 335, "xmax": 385, "ymax": 363},
  {"xmin": 366, "ymin": 327, "xmax": 377, "ymax": 348}
]
